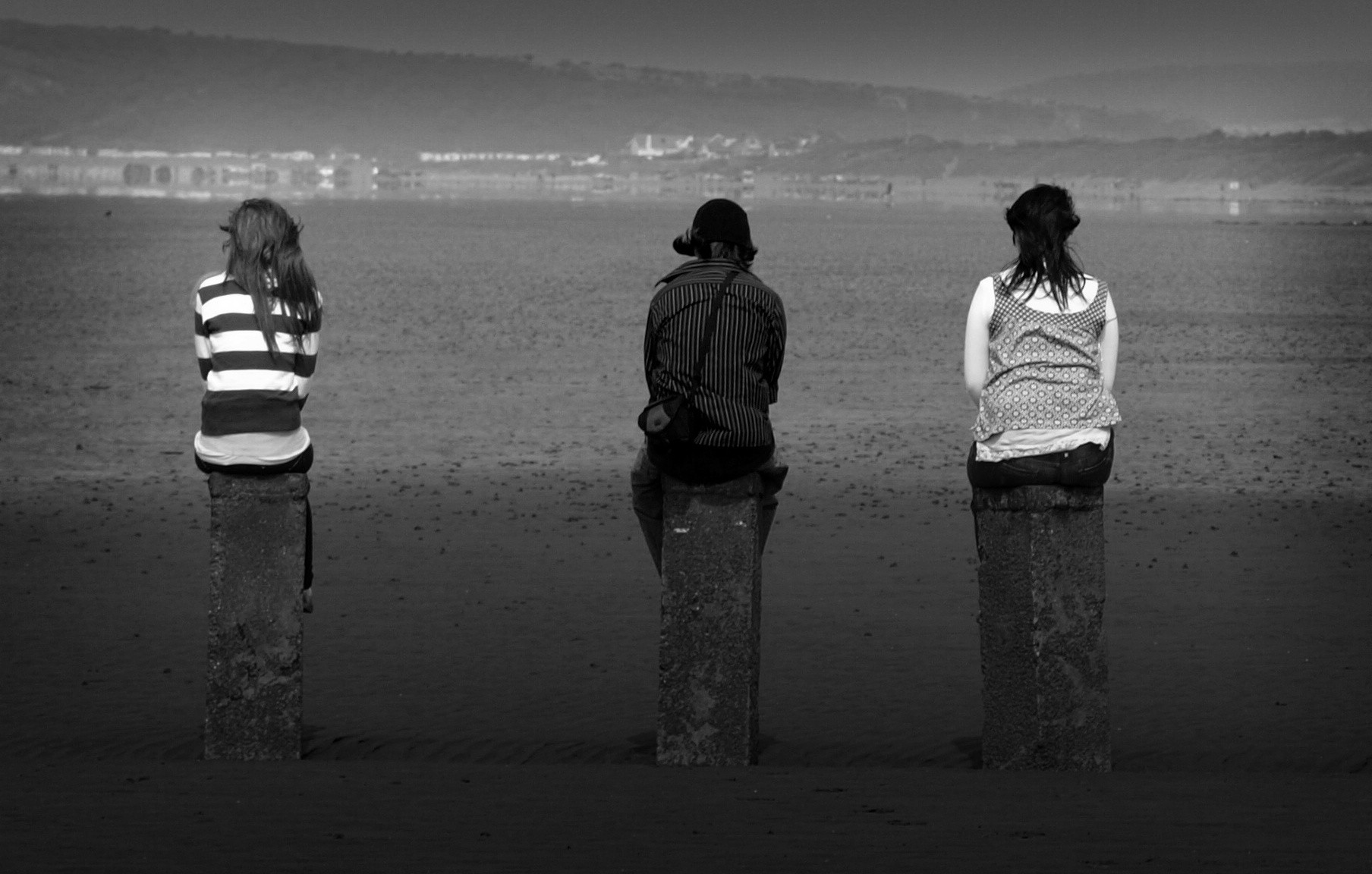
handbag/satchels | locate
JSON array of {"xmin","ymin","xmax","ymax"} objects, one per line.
[{"xmin": 636, "ymin": 394, "xmax": 701, "ymax": 455}]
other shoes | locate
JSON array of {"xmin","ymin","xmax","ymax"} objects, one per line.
[{"xmin": 299, "ymin": 581, "xmax": 313, "ymax": 614}]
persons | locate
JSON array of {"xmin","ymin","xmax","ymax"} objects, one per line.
[
  {"xmin": 964, "ymin": 185, "xmax": 1121, "ymax": 564},
  {"xmin": 632, "ymin": 199, "xmax": 788, "ymax": 578},
  {"xmin": 196, "ymin": 197, "xmax": 322, "ymax": 610}
]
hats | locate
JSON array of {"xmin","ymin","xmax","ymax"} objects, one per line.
[{"xmin": 672, "ymin": 197, "xmax": 758, "ymax": 264}]
